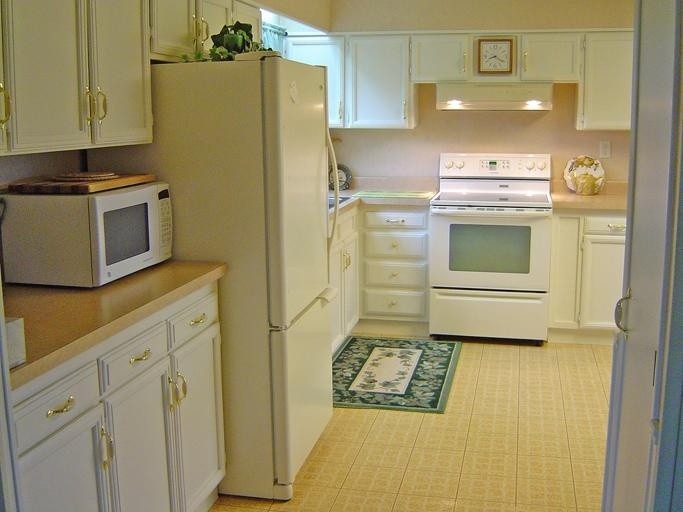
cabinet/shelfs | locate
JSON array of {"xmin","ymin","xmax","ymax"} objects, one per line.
[
  {"xmin": 283, "ymin": 32, "xmax": 347, "ymax": 129},
  {"xmin": 0, "ymin": 345, "xmax": 114, "ymax": 512},
  {"xmin": 409, "ymin": 30, "xmax": 473, "ymax": 84},
  {"xmin": 0, "ymin": 1, "xmax": 154, "ymax": 157},
  {"xmin": 550, "ymin": 212, "xmax": 628, "ymax": 330},
  {"xmin": 99, "ymin": 278, "xmax": 228, "ymax": 512},
  {"xmin": 513, "ymin": 31, "xmax": 582, "ymax": 84},
  {"xmin": 327, "ymin": 204, "xmax": 359, "ymax": 359},
  {"xmin": 347, "ymin": 30, "xmax": 418, "ymax": 129},
  {"xmin": 574, "ymin": 28, "xmax": 633, "ymax": 129},
  {"xmin": 231, "ymin": 0, "xmax": 261, "ymax": 43},
  {"xmin": 359, "ymin": 206, "xmax": 428, "ymax": 323},
  {"xmin": 145, "ymin": 0, "xmax": 232, "ymax": 61}
]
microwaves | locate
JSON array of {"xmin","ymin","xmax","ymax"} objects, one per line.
[{"xmin": 1, "ymin": 182, "xmax": 175, "ymax": 288}]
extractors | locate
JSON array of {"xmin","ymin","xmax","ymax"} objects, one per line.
[{"xmin": 433, "ymin": 80, "xmax": 554, "ymax": 112}]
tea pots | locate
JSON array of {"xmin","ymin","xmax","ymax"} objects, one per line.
[{"xmin": 569, "ymin": 171, "xmax": 606, "ymax": 196}]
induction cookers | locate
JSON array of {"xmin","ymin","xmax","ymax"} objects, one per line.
[{"xmin": 429, "ymin": 191, "xmax": 553, "ymax": 208}]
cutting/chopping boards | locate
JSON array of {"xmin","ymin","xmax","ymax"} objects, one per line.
[{"xmin": 6, "ymin": 174, "xmax": 157, "ymax": 194}]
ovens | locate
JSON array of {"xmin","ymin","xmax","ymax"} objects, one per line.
[{"xmin": 428, "ymin": 206, "xmax": 553, "ymax": 343}]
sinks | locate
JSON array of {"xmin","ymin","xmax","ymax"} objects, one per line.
[{"xmin": 328, "ymin": 196, "xmax": 350, "ymax": 211}]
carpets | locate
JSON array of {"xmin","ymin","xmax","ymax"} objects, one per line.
[{"xmin": 331, "ymin": 335, "xmax": 462, "ymax": 413}]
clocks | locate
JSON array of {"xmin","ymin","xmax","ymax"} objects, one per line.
[{"xmin": 478, "ymin": 38, "xmax": 513, "ymax": 74}]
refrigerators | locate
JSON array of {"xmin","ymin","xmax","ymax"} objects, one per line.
[{"xmin": 99, "ymin": 61, "xmax": 339, "ymax": 503}]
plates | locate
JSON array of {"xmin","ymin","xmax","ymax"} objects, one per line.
[
  {"xmin": 328, "ymin": 165, "xmax": 352, "ymax": 190},
  {"xmin": 561, "ymin": 154, "xmax": 604, "ymax": 190}
]
